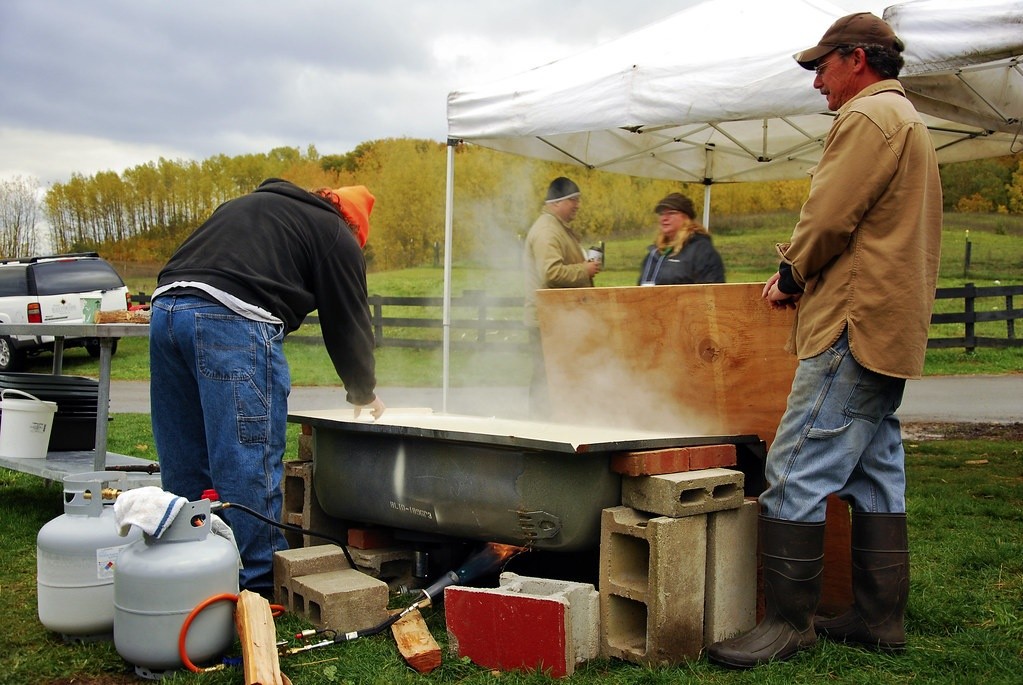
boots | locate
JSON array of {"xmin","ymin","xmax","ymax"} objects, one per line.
[
  {"xmin": 709, "ymin": 513, "xmax": 827, "ymax": 669},
  {"xmin": 814, "ymin": 510, "xmax": 910, "ymax": 654}
]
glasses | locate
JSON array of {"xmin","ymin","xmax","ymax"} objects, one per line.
[
  {"xmin": 657, "ymin": 211, "xmax": 679, "ymax": 217},
  {"xmin": 568, "ymin": 197, "xmax": 580, "ymax": 203},
  {"xmin": 813, "ymin": 54, "xmax": 850, "ymax": 77}
]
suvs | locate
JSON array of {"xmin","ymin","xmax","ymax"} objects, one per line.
[{"xmin": 0, "ymin": 252, "xmax": 132, "ymax": 372}]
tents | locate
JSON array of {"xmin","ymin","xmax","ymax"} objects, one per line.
[{"xmin": 444, "ymin": 0, "xmax": 1023, "ymax": 415}]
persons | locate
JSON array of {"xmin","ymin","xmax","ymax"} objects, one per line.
[
  {"xmin": 706, "ymin": 13, "xmax": 944, "ymax": 670},
  {"xmin": 522, "ymin": 176, "xmax": 602, "ymax": 423},
  {"xmin": 152, "ymin": 177, "xmax": 386, "ymax": 605},
  {"xmin": 636, "ymin": 192, "xmax": 726, "ymax": 286}
]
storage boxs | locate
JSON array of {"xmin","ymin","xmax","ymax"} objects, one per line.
[{"xmin": 0, "ymin": 372, "xmax": 114, "ymax": 453}]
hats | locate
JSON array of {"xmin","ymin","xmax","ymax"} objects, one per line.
[
  {"xmin": 318, "ymin": 185, "xmax": 376, "ymax": 249},
  {"xmin": 655, "ymin": 192, "xmax": 697, "ymax": 220},
  {"xmin": 543, "ymin": 177, "xmax": 582, "ymax": 203},
  {"xmin": 791, "ymin": 11, "xmax": 902, "ymax": 70}
]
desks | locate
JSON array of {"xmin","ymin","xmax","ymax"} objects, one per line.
[{"xmin": 0, "ymin": 322, "xmax": 162, "ymax": 492}]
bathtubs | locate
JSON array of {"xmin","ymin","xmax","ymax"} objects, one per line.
[{"xmin": 286, "ymin": 406, "xmax": 768, "ymax": 555}]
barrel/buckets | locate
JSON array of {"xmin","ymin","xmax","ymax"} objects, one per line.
[{"xmin": 0, "ymin": 388, "xmax": 58, "ymax": 459}]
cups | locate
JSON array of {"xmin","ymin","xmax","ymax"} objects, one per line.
[
  {"xmin": 588, "ymin": 245, "xmax": 603, "ymax": 263},
  {"xmin": 79, "ymin": 296, "xmax": 102, "ymax": 321}
]
